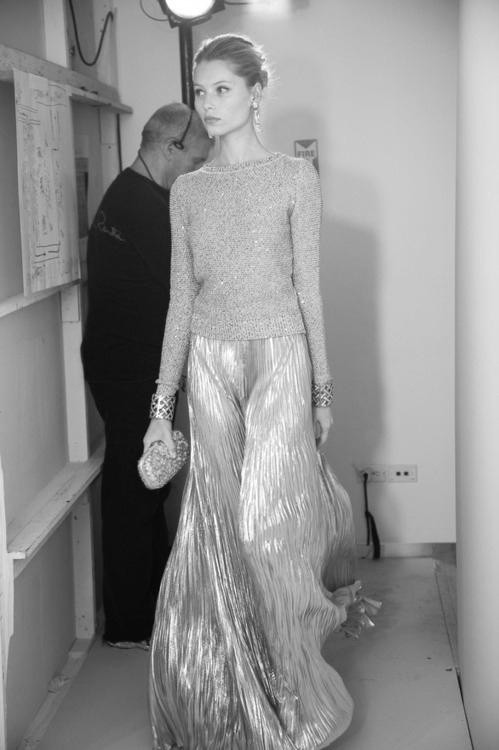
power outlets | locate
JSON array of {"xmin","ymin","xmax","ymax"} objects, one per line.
[{"xmin": 355, "ymin": 464, "xmax": 385, "ymax": 483}]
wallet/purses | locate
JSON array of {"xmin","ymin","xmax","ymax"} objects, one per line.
[{"xmin": 136, "ymin": 429, "xmax": 191, "ymax": 491}]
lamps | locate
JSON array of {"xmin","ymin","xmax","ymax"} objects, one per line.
[{"xmin": 157, "ymin": 0, "xmax": 217, "ymax": 110}]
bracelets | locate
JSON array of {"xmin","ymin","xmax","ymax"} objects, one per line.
[
  {"xmin": 311, "ymin": 379, "xmax": 337, "ymax": 408},
  {"xmin": 149, "ymin": 393, "xmax": 177, "ymax": 421}
]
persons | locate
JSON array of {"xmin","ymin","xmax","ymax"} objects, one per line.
[
  {"xmin": 81, "ymin": 101, "xmax": 214, "ymax": 649},
  {"xmin": 143, "ymin": 31, "xmax": 385, "ymax": 750}
]
